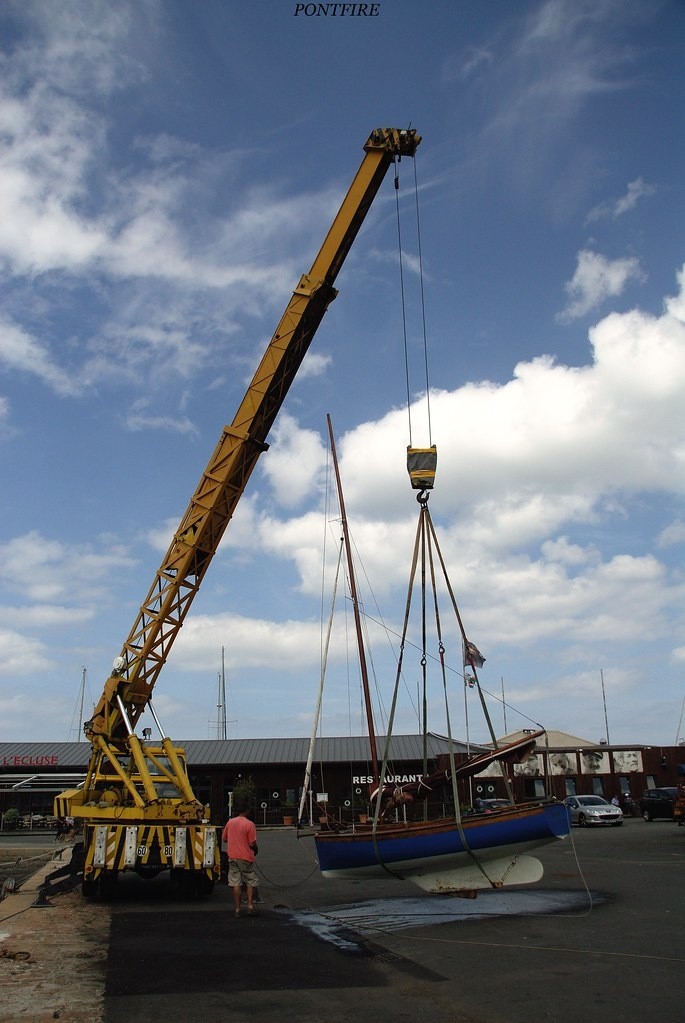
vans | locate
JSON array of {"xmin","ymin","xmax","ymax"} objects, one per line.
[{"xmin": 640, "ymin": 787, "xmax": 685, "ymax": 822}]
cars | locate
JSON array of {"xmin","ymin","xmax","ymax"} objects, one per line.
[{"xmin": 563, "ymin": 795, "xmax": 624, "ymax": 827}]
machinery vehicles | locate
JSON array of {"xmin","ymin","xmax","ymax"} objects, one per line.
[{"xmin": 30, "ymin": 124, "xmax": 435, "ymax": 907}]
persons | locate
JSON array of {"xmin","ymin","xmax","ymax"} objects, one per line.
[
  {"xmin": 611, "ymin": 795, "xmax": 620, "ymax": 806},
  {"xmin": 613, "ymin": 751, "xmax": 638, "ymax": 773},
  {"xmin": 580, "ymin": 752, "xmax": 603, "ymax": 774},
  {"xmin": 54, "ymin": 816, "xmax": 69, "ymax": 841},
  {"xmin": 549, "ymin": 754, "xmax": 575, "ymax": 774},
  {"xmin": 516, "ymin": 754, "xmax": 542, "ymax": 776},
  {"xmin": 222, "ymin": 801, "xmax": 258, "ymax": 916},
  {"xmin": 624, "ymin": 793, "xmax": 635, "ymax": 815}
]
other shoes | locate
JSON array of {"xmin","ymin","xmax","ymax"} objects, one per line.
[
  {"xmin": 246, "ymin": 907, "xmax": 256, "ymax": 915},
  {"xmin": 234, "ymin": 911, "xmax": 241, "ymax": 917}
]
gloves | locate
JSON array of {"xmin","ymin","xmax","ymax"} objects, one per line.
[{"xmin": 250, "ymin": 843, "xmax": 258, "ymax": 856}]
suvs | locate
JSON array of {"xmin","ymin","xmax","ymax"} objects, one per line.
[{"xmin": 468, "ymin": 799, "xmax": 518, "ymax": 814}]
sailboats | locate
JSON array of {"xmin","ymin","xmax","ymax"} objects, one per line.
[{"xmin": 296, "ymin": 413, "xmax": 571, "ymax": 899}]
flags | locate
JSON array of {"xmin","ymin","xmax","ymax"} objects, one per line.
[{"xmin": 464, "ymin": 641, "xmax": 486, "ymax": 668}]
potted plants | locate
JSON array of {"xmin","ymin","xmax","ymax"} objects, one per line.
[
  {"xmin": 281, "ymin": 800, "xmax": 293, "ymax": 825},
  {"xmin": 356, "ymin": 798, "xmax": 370, "ymax": 824},
  {"xmin": 319, "ymin": 800, "xmax": 328, "ymax": 823}
]
